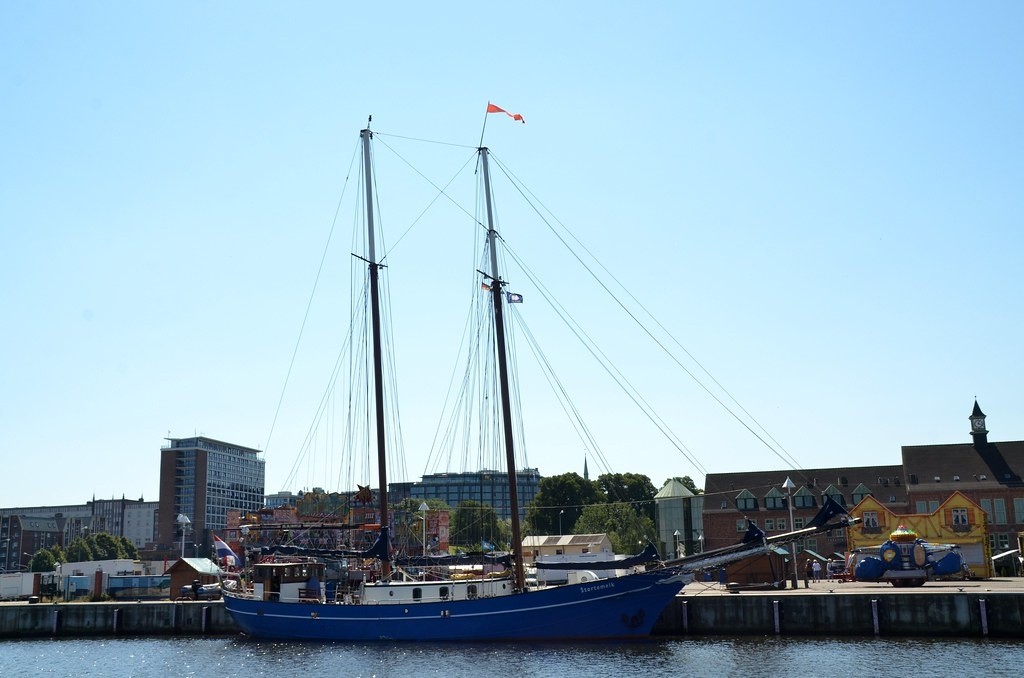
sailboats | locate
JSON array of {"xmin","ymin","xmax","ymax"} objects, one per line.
[{"xmin": 218, "ymin": 111, "xmax": 868, "ymax": 644}]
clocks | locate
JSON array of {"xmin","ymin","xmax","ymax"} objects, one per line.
[{"xmin": 974, "ymin": 420, "xmax": 984, "ymax": 428}]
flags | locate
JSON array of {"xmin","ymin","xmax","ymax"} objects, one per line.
[
  {"xmin": 488, "ymin": 102, "xmax": 526, "ymax": 124},
  {"xmin": 482, "ymin": 540, "xmax": 495, "ymax": 551},
  {"xmin": 507, "ymin": 291, "xmax": 523, "ymax": 304},
  {"xmin": 215, "ymin": 534, "xmax": 240, "ymax": 565}
]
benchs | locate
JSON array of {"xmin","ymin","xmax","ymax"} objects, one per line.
[{"xmin": 298, "ymin": 588, "xmax": 321, "ymax": 603}]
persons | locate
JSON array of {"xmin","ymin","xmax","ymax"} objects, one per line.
[
  {"xmin": 826, "ymin": 558, "xmax": 834, "ymax": 582},
  {"xmin": 812, "ymin": 559, "xmax": 822, "ymax": 583},
  {"xmin": 805, "ymin": 559, "xmax": 814, "ymax": 583}
]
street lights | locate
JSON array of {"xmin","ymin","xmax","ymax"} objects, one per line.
[
  {"xmin": 179, "ymin": 513, "xmax": 191, "ymax": 558},
  {"xmin": 673, "ymin": 529, "xmax": 680, "ymax": 560},
  {"xmin": 560, "ymin": 509, "xmax": 563, "ymax": 535},
  {"xmin": 417, "ymin": 500, "xmax": 430, "ymax": 557},
  {"xmin": 781, "ymin": 477, "xmax": 799, "ymax": 583},
  {"xmin": 697, "ymin": 535, "xmax": 704, "ymax": 555}
]
